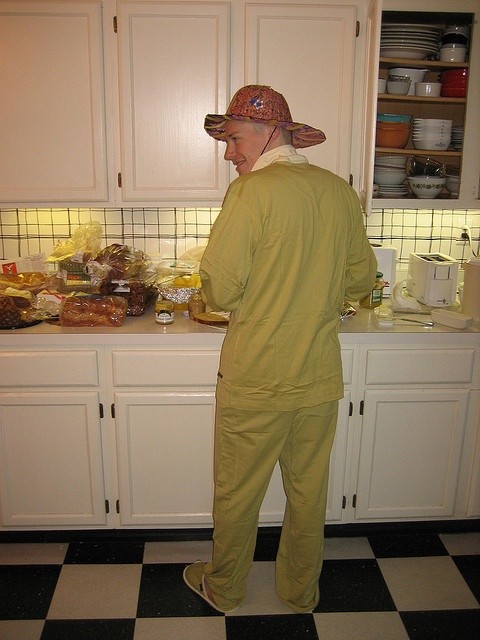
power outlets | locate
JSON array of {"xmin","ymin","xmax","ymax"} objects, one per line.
[{"xmin": 455, "ymin": 218, "xmax": 474, "ymax": 245}]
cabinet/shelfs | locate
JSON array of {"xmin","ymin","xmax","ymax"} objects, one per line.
[
  {"xmin": 0, "ymin": 0, "xmax": 108, "ymax": 209},
  {"xmin": 258, "ymin": 333, "xmax": 354, "ymax": 527},
  {"xmin": 111, "ymin": 334, "xmax": 218, "ymax": 530},
  {"xmin": 362, "ymin": 1, "xmax": 480, "ymax": 216},
  {"xmin": 354, "ymin": 333, "xmax": 479, "ymax": 520},
  {"xmin": 116, "ymin": 2, "xmax": 355, "ymax": 208},
  {"xmin": 0, "ymin": 334, "xmax": 106, "ymax": 531}
]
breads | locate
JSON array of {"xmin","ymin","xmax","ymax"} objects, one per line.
[
  {"xmin": 92, "ymin": 243, "xmax": 153, "ymax": 280},
  {"xmin": 99, "ymin": 272, "xmax": 158, "ymax": 316},
  {"xmin": 60, "ymin": 296, "xmax": 128, "ymax": 327},
  {"xmin": 0, "ymin": 272, "xmax": 45, "ymax": 298},
  {"xmin": 6, "ymin": 287, "xmax": 38, "ymax": 309},
  {"xmin": 194, "ymin": 313, "xmax": 230, "ymax": 325}
]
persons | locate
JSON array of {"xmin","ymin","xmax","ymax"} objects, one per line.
[{"xmin": 177, "ymin": 85, "xmax": 378, "ymax": 612}]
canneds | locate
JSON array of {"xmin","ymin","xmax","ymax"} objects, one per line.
[{"xmin": 189, "ymin": 292, "xmax": 206, "ymax": 320}]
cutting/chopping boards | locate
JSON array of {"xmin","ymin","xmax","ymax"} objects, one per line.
[{"xmin": 192, "ymin": 313, "xmax": 229, "ymax": 326}]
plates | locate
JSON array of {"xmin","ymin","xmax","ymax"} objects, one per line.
[
  {"xmin": 450, "ymin": 127, "xmax": 463, "ymax": 152},
  {"xmin": 441, "ymin": 68, "xmax": 468, "ymax": 98},
  {"xmin": 380, "ymin": 23, "xmax": 441, "ymax": 60},
  {"xmin": 378, "ymin": 184, "xmax": 409, "ymax": 197}
]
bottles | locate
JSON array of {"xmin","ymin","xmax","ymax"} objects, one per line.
[{"xmin": 188, "ymin": 288, "xmax": 206, "ymax": 320}]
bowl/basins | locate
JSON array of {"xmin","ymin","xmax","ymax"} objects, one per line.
[
  {"xmin": 445, "ymin": 175, "xmax": 460, "ymax": 196},
  {"xmin": 375, "ymin": 113, "xmax": 412, "ymax": 148},
  {"xmin": 378, "ymin": 79, "xmax": 386, "ymax": 94},
  {"xmin": 389, "ymin": 68, "xmax": 428, "ymax": 95},
  {"xmin": 374, "ymin": 155, "xmax": 409, "ymax": 185},
  {"xmin": 406, "ymin": 175, "xmax": 446, "ymax": 198},
  {"xmin": 386, "ymin": 75, "xmax": 412, "ymax": 95},
  {"xmin": 414, "ymin": 82, "xmax": 442, "ymax": 98},
  {"xmin": 412, "ymin": 118, "xmax": 453, "ymax": 151},
  {"xmin": 437, "ymin": 43, "xmax": 467, "ymax": 63}
]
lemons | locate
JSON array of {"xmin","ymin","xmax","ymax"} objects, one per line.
[{"xmin": 173, "ymin": 277, "xmax": 188, "ymax": 285}]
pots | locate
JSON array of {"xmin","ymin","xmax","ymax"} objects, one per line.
[{"xmin": 409, "ymin": 155, "xmax": 444, "ymax": 180}]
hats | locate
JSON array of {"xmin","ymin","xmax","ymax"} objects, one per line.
[{"xmin": 204, "ymin": 85, "xmax": 326, "ymax": 149}]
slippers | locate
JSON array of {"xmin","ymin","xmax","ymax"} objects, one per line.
[{"xmin": 182, "ymin": 560, "xmax": 239, "ymax": 613}]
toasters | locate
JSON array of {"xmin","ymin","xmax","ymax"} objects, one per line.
[{"xmin": 405, "ymin": 252, "xmax": 459, "ymax": 308}]
